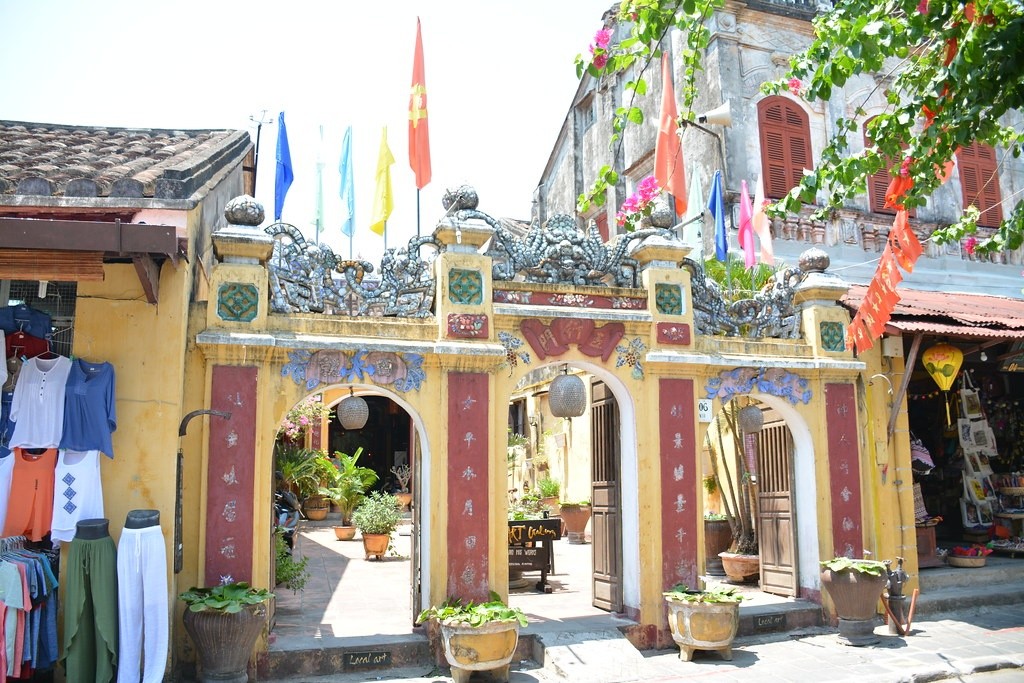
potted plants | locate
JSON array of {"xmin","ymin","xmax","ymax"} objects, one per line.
[
  {"xmin": 819, "ymin": 557, "xmax": 889, "ymax": 647},
  {"xmin": 662, "ymin": 585, "xmax": 743, "ymax": 662},
  {"xmin": 705, "ymin": 257, "xmax": 787, "ymax": 584},
  {"xmin": 416, "ymin": 590, "xmax": 528, "ymax": 683},
  {"xmin": 537, "ymin": 479, "xmax": 591, "ymax": 543},
  {"xmin": 179, "ymin": 581, "xmax": 274, "ymax": 683},
  {"xmin": 276, "ymin": 445, "xmax": 412, "ymax": 560}
]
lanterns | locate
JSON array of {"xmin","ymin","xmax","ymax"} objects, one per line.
[{"xmin": 922, "ymin": 338, "xmax": 963, "ymax": 428}]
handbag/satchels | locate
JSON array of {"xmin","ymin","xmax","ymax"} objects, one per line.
[
  {"xmin": 912, "ymin": 483, "xmax": 928, "ymax": 520},
  {"xmin": 956, "ymin": 370, "xmax": 999, "ymax": 527},
  {"xmin": 909, "ymin": 430, "xmax": 932, "ymax": 475}
]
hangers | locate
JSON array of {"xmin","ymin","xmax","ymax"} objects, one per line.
[
  {"xmin": 1, "ymin": 291, "xmax": 105, "ymax": 407},
  {"xmin": 0, "ymin": 535, "xmax": 32, "ymax": 562}
]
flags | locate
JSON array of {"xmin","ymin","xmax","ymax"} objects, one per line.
[
  {"xmin": 367, "ymin": 125, "xmax": 395, "ymax": 237},
  {"xmin": 337, "ymin": 124, "xmax": 355, "ymax": 237},
  {"xmin": 738, "ymin": 177, "xmax": 756, "ymax": 273},
  {"xmin": 653, "ymin": 50, "xmax": 687, "ymax": 217},
  {"xmin": 844, "ymin": 1, "xmax": 993, "ymax": 357},
  {"xmin": 408, "ymin": 17, "xmax": 432, "ymax": 190},
  {"xmin": 314, "ymin": 121, "xmax": 324, "ymax": 233},
  {"xmin": 709, "ymin": 169, "xmax": 729, "ymax": 262},
  {"xmin": 275, "ymin": 112, "xmax": 295, "ymax": 220}
]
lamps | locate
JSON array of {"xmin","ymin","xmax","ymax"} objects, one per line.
[{"xmin": 681, "ymin": 100, "xmax": 733, "ymax": 188}]
